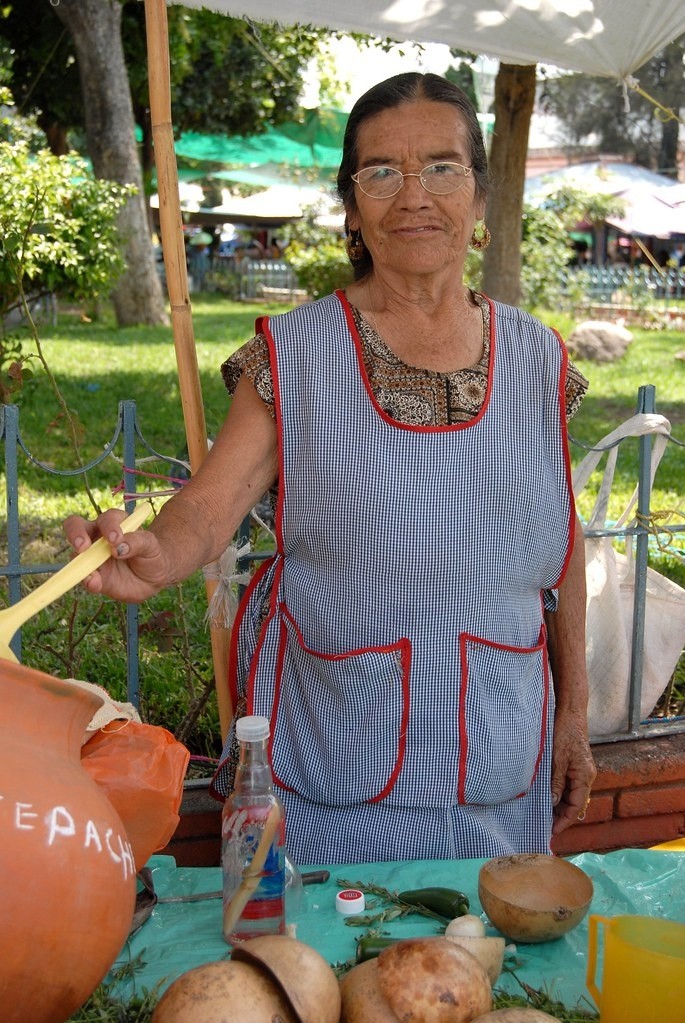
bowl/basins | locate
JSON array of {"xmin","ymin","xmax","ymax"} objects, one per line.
[{"xmin": 478, "ymin": 854, "xmax": 594, "ymax": 942}]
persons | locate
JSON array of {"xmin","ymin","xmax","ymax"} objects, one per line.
[
  {"xmin": 63, "ymin": 70, "xmax": 597, "ymax": 866},
  {"xmin": 567, "ymin": 239, "xmax": 589, "ymax": 267}
]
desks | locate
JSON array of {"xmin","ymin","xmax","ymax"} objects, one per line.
[{"xmin": 68, "ymin": 848, "xmax": 685, "ymax": 1023}]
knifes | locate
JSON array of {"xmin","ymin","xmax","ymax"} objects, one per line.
[{"xmin": 158, "ymin": 868, "xmax": 329, "ymax": 904}]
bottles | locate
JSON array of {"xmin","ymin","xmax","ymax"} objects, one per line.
[{"xmin": 221, "ymin": 717, "xmax": 286, "ymax": 942}]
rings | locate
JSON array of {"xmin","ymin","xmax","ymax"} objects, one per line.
[{"xmin": 577, "ymin": 812, "xmax": 586, "ymax": 820}]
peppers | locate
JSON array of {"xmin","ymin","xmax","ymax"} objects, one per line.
[
  {"xmin": 356, "ymin": 937, "xmax": 408, "ymax": 962},
  {"xmin": 397, "ymin": 887, "xmax": 470, "ymax": 920}
]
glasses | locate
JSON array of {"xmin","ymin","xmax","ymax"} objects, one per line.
[{"xmin": 350, "ymin": 161, "xmax": 477, "ymax": 199}]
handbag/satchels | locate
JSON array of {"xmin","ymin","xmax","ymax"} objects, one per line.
[{"xmin": 572, "ymin": 413, "xmax": 685, "ymax": 735}]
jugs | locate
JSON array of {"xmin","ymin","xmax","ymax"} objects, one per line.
[{"xmin": 587, "ymin": 914, "xmax": 685, "ymax": 1023}]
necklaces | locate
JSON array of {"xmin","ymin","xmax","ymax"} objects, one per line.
[{"xmin": 366, "ymin": 283, "xmax": 382, "ymax": 339}]
